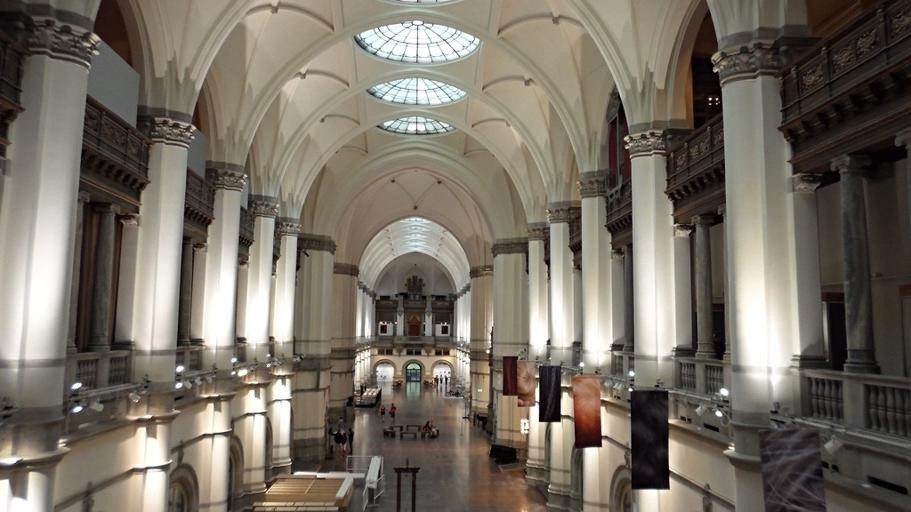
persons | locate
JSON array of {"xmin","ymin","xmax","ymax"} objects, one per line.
[
  {"xmin": 428, "ymin": 421, "xmax": 438, "ymax": 438},
  {"xmin": 388, "ymin": 402, "xmax": 397, "ymax": 426},
  {"xmin": 331, "ymin": 416, "xmax": 355, "ymax": 456},
  {"xmin": 422, "ymin": 420, "xmax": 433, "ymax": 440},
  {"xmin": 379, "ymin": 404, "xmax": 388, "ymax": 422}
]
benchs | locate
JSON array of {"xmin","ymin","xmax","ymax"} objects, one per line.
[{"xmin": 384, "ymin": 424, "xmax": 439, "ymax": 440}]
